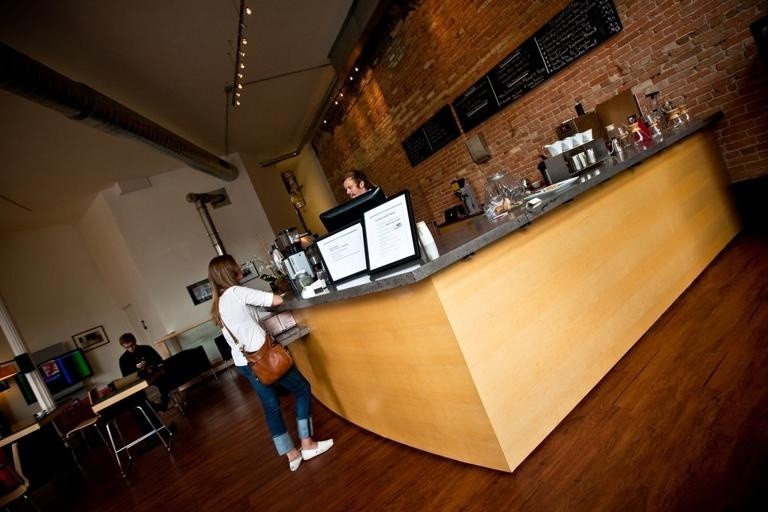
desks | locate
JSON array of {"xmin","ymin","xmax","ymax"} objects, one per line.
[{"xmin": 152, "ymin": 317, "xmax": 235, "ymax": 392}]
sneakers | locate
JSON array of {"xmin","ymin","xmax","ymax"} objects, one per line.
[
  {"xmin": 289, "ymin": 456, "xmax": 303, "ymax": 473},
  {"xmin": 302, "ymin": 439, "xmax": 334, "ymax": 461}
]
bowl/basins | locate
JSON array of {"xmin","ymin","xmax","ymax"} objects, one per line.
[
  {"xmin": 530, "ymin": 180, "xmax": 542, "ymax": 189},
  {"xmin": 544, "ymin": 127, "xmax": 593, "ymax": 157}
]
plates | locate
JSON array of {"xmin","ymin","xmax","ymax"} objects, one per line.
[
  {"xmin": 524, "ymin": 176, "xmax": 580, "ymax": 202},
  {"xmin": 35, "ymin": 412, "xmax": 48, "ymax": 419}
]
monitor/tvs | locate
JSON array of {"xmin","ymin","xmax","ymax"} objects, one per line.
[
  {"xmin": 318, "ymin": 186, "xmax": 387, "ymax": 235},
  {"xmin": 13, "ymin": 347, "xmax": 94, "ymax": 405}
]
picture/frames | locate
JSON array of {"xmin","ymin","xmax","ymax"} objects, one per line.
[
  {"xmin": 70, "ymin": 325, "xmax": 110, "ymax": 352},
  {"xmin": 236, "ymin": 260, "xmax": 259, "ymax": 286},
  {"xmin": 312, "ymin": 219, "xmax": 369, "ymax": 286},
  {"xmin": 359, "ymin": 188, "xmax": 422, "ymax": 276},
  {"xmin": 185, "ymin": 278, "xmax": 213, "ymax": 305}
]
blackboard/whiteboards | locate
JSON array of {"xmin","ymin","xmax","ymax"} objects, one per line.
[
  {"xmin": 400, "ymin": 103, "xmax": 463, "ymax": 167},
  {"xmin": 450, "ymin": 0, "xmax": 622, "ymax": 133}
]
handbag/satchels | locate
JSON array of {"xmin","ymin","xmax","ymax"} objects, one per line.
[{"xmin": 241, "ymin": 333, "xmax": 294, "ymax": 386}]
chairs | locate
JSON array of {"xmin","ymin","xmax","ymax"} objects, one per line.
[{"xmin": 0, "ymin": 334, "xmax": 233, "ymax": 512}]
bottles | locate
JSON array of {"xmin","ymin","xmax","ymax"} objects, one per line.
[
  {"xmin": 611, "ymin": 101, "xmax": 694, "ymax": 155},
  {"xmin": 483, "ymin": 165, "xmax": 526, "ymax": 217}
]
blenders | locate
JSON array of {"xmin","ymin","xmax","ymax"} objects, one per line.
[{"xmin": 273, "ymin": 226, "xmax": 322, "ymax": 292}]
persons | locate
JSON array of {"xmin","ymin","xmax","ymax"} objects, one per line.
[
  {"xmin": 208, "ymin": 255, "xmax": 334, "ymax": 472},
  {"xmin": 118, "ymin": 332, "xmax": 171, "ymax": 413},
  {"xmin": 341, "ymin": 166, "xmax": 388, "ymax": 200}
]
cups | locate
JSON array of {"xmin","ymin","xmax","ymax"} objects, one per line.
[
  {"xmin": 415, "ymin": 221, "xmax": 436, "ymax": 249},
  {"xmin": 36, "ymin": 410, "xmax": 46, "ymax": 416}
]
